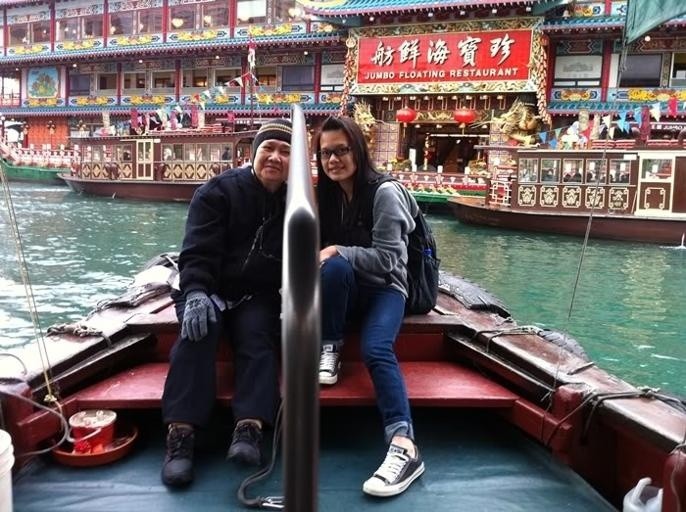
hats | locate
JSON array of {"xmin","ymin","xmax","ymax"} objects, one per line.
[{"xmin": 252, "ymin": 118, "xmax": 292, "ymax": 158}]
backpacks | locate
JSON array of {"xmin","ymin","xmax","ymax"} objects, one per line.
[{"xmin": 362, "ymin": 177, "xmax": 437, "ymax": 315}]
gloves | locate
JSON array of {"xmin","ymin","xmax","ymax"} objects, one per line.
[{"xmin": 181, "ymin": 290, "xmax": 217, "ymax": 341}]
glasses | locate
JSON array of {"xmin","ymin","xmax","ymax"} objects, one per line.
[{"xmin": 318, "ymin": 146, "xmax": 353, "ymax": 158}]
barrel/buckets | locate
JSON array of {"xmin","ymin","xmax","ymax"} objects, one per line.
[
  {"xmin": 0, "ymin": 428, "xmax": 16, "ymax": 512},
  {"xmin": 66, "ymin": 408, "xmax": 118, "ymax": 454}
]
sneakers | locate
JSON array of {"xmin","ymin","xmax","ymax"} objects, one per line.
[
  {"xmin": 318, "ymin": 340, "xmax": 343, "ymax": 385},
  {"xmin": 363, "ymin": 443, "xmax": 425, "ymax": 497},
  {"xmin": 226, "ymin": 422, "xmax": 262, "ymax": 466},
  {"xmin": 162, "ymin": 427, "xmax": 194, "ymax": 488}
]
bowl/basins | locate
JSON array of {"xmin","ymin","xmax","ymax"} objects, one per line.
[{"xmin": 49, "ymin": 423, "xmax": 139, "ymax": 468}]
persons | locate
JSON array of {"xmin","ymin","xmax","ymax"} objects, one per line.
[
  {"xmin": 160, "ymin": 117, "xmax": 292, "ymax": 487},
  {"xmin": 314, "ymin": 114, "xmax": 425, "ymax": 498}
]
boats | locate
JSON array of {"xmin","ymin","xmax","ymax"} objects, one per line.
[
  {"xmin": 53, "ymin": 69, "xmax": 264, "ymax": 206},
  {"xmin": 0, "ymin": 248, "xmax": 686, "ymax": 511},
  {"xmin": 442, "ymin": 93, "xmax": 686, "ymax": 247}
]
